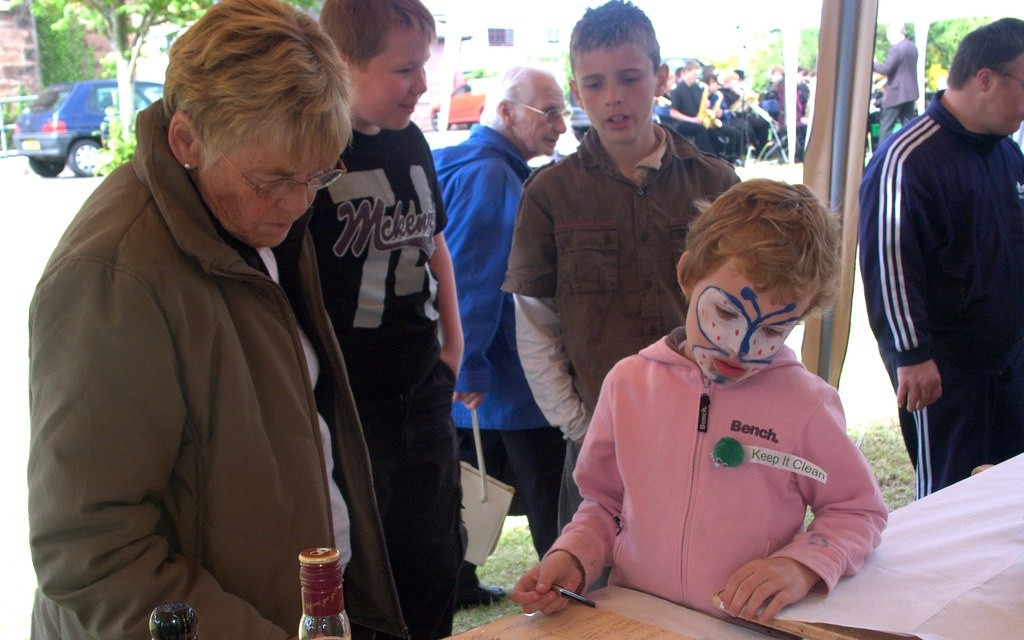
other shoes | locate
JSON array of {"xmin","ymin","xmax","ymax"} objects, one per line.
[{"xmin": 454, "ymin": 586, "xmax": 506, "ymax": 609}]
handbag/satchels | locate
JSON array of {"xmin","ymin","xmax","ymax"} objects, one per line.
[{"xmin": 452, "ymin": 400, "xmax": 515, "ymax": 566}]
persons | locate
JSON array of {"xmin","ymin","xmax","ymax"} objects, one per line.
[
  {"xmin": 566, "ymin": 17, "xmax": 920, "ymax": 167},
  {"xmin": 309, "ymin": 0, "xmax": 466, "ymax": 640},
  {"xmin": 431, "ymin": 64, "xmax": 566, "ymax": 608},
  {"xmin": 858, "ymin": 17, "xmax": 1024, "ymax": 503},
  {"xmin": 502, "ymin": 0, "xmax": 742, "ymax": 592},
  {"xmin": 27, "ymin": 0, "xmax": 409, "ymax": 640},
  {"xmin": 508, "ymin": 178, "xmax": 888, "ymax": 623}
]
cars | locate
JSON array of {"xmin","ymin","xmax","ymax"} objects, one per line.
[
  {"xmin": 11, "ymin": 80, "xmax": 163, "ymax": 179},
  {"xmin": 431, "ymin": 76, "xmax": 503, "ymax": 133}
]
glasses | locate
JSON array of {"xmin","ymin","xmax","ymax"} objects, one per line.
[
  {"xmin": 507, "ymin": 98, "xmax": 573, "ymax": 125},
  {"xmin": 217, "ymin": 150, "xmax": 346, "ymax": 199}
]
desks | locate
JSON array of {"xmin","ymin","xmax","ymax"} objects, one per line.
[
  {"xmin": 716, "ymin": 467, "xmax": 1024, "ymax": 640},
  {"xmin": 441, "ymin": 586, "xmax": 779, "ymax": 640}
]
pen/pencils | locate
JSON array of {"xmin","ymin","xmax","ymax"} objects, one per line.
[{"xmin": 550, "ymin": 583, "xmax": 599, "ymax": 609}]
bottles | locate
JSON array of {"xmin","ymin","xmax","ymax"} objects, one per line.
[
  {"xmin": 149, "ymin": 601, "xmax": 199, "ymax": 640},
  {"xmin": 298, "ymin": 547, "xmax": 352, "ymax": 640}
]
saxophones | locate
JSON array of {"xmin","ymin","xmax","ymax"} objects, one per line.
[
  {"xmin": 711, "ymin": 92, "xmax": 725, "ymax": 129},
  {"xmin": 696, "ymin": 78, "xmax": 717, "ymax": 130}
]
